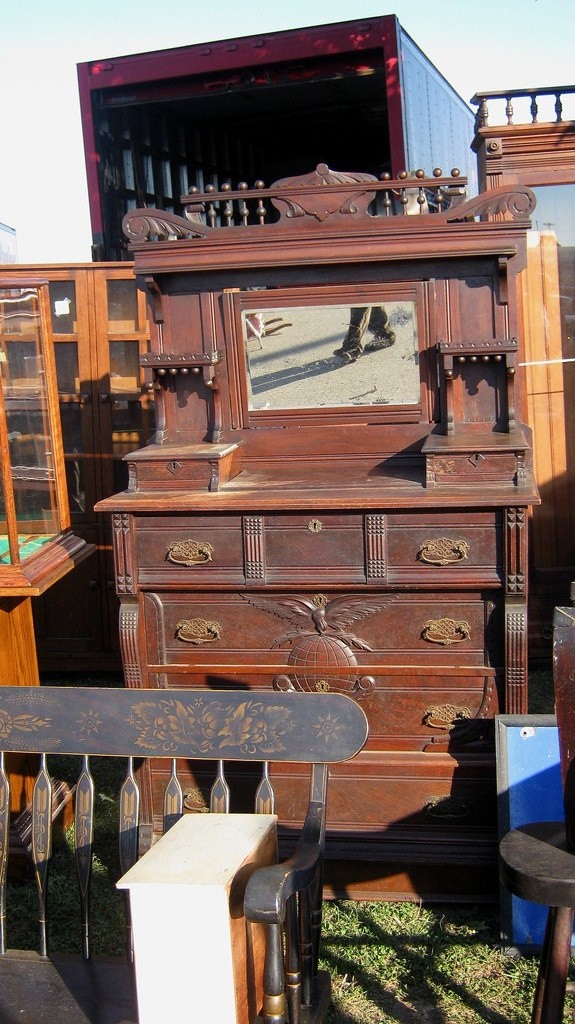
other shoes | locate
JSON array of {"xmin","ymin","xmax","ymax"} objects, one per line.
[{"xmin": 333, "ymin": 332, "xmax": 395, "ymax": 361}]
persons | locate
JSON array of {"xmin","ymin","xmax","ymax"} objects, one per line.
[{"xmin": 332, "ymin": 307, "xmax": 396, "ymax": 359}]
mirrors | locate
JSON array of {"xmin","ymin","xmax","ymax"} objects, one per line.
[{"xmin": 220, "ymin": 283, "xmax": 428, "ymax": 431}]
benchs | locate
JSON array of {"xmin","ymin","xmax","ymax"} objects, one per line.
[{"xmin": 1, "ymin": 685, "xmax": 370, "ymax": 1024}]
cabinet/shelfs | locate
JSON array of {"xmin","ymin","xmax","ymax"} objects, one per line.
[
  {"xmin": 0, "ymin": 260, "xmax": 154, "ymax": 548},
  {"xmin": 94, "ymin": 187, "xmax": 542, "ymax": 928}
]
